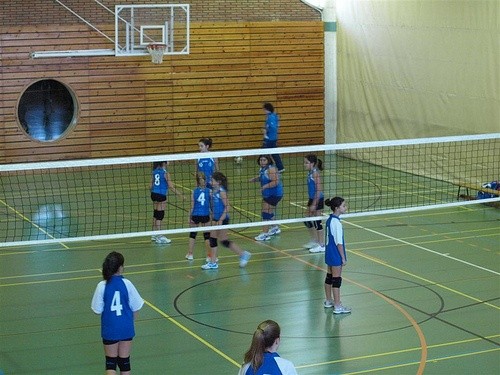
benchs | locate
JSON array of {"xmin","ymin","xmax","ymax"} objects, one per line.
[{"xmin": 451, "ymin": 179, "xmax": 500, "ymax": 201}]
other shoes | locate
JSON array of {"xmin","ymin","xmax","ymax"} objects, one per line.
[{"xmin": 278, "ymin": 167, "xmax": 285, "ymax": 173}]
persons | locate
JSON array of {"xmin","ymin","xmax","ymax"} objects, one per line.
[
  {"xmin": 186, "ymin": 172, "xmax": 218, "ymax": 262},
  {"xmin": 324, "ymin": 197, "xmax": 352, "ymax": 314},
  {"xmin": 259, "ymin": 103, "xmax": 285, "ymax": 173},
  {"xmin": 246, "ymin": 155, "xmax": 283, "ymax": 241},
  {"xmin": 201, "ymin": 172, "xmax": 251, "ymax": 270},
  {"xmin": 91, "ymin": 252, "xmax": 144, "ymax": 375},
  {"xmin": 238, "ymin": 320, "xmax": 297, "ymax": 375},
  {"xmin": 195, "ymin": 138, "xmax": 218, "ymax": 190},
  {"xmin": 149, "ymin": 161, "xmax": 187, "ymax": 244},
  {"xmin": 302, "ymin": 154, "xmax": 325, "ymax": 252},
  {"xmin": 476, "ymin": 181, "xmax": 500, "ymax": 206}
]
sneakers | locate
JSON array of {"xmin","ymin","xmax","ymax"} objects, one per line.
[
  {"xmin": 206, "ymin": 256, "xmax": 219, "ymax": 262},
  {"xmin": 255, "ymin": 233, "xmax": 270, "ymax": 240},
  {"xmin": 202, "ymin": 262, "xmax": 218, "ymax": 269},
  {"xmin": 302, "ymin": 241, "xmax": 317, "ymax": 248},
  {"xmin": 268, "ymin": 226, "xmax": 281, "ymax": 235},
  {"xmin": 333, "ymin": 304, "xmax": 352, "ymax": 313},
  {"xmin": 309, "ymin": 244, "xmax": 326, "ymax": 252},
  {"xmin": 151, "ymin": 236, "xmax": 156, "ymax": 240},
  {"xmin": 324, "ymin": 299, "xmax": 334, "ymax": 307},
  {"xmin": 156, "ymin": 236, "xmax": 171, "ymax": 243},
  {"xmin": 186, "ymin": 253, "xmax": 193, "ymax": 259},
  {"xmin": 240, "ymin": 251, "xmax": 251, "ymax": 267}
]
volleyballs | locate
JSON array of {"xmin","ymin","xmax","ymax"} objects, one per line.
[{"xmin": 234, "ymin": 156, "xmax": 243, "ymax": 165}]
